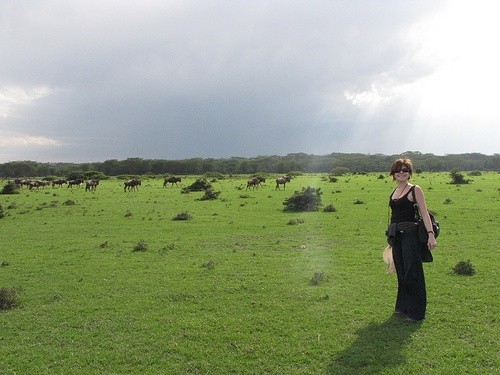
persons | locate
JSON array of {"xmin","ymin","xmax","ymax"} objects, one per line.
[{"xmin": 380, "ymin": 157, "xmax": 437, "ymax": 323}]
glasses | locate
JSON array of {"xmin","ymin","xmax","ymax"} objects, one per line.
[{"xmin": 394, "ymin": 167, "xmax": 409, "ymax": 173}]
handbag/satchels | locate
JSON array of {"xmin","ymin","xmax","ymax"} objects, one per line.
[{"xmin": 416, "ymin": 212, "xmax": 441, "ymax": 241}]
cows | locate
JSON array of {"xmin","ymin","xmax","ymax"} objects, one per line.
[
  {"xmin": 123, "ymin": 178, "xmax": 142, "ymax": 192},
  {"xmin": 246, "ymin": 176, "xmax": 291, "ymax": 191},
  {"xmin": 20, "ymin": 177, "xmax": 100, "ymax": 192},
  {"xmin": 163, "ymin": 177, "xmax": 182, "ymax": 188}
]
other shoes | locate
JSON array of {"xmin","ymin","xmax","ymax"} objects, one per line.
[{"xmin": 394, "ymin": 311, "xmax": 419, "ymax": 324}]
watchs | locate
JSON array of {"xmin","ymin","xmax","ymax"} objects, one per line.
[{"xmin": 427, "ymin": 230, "xmax": 436, "ymax": 235}]
